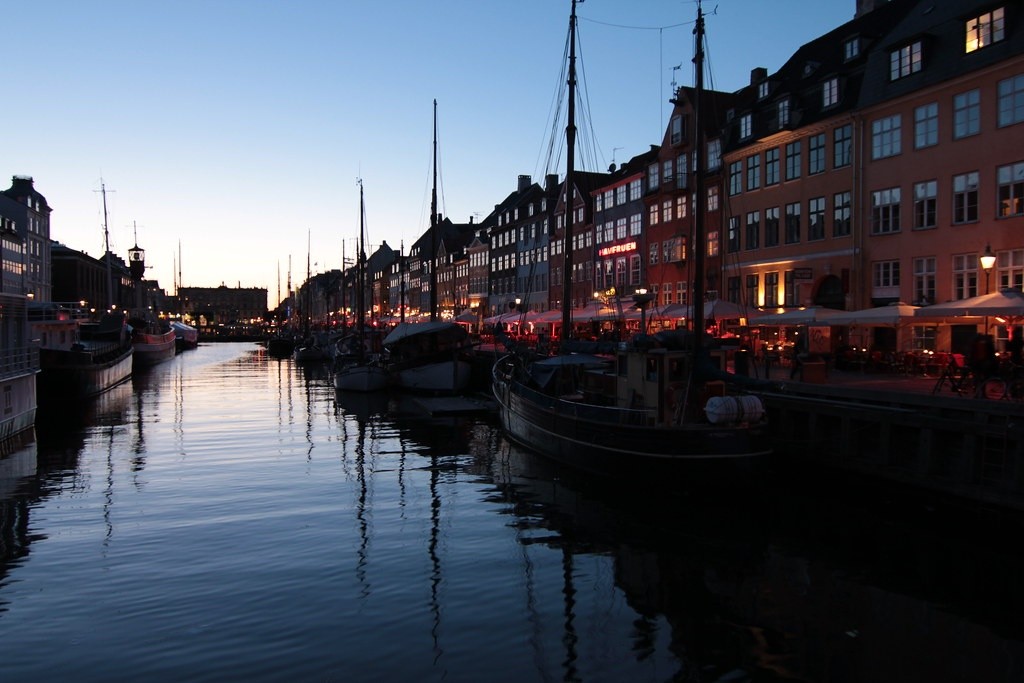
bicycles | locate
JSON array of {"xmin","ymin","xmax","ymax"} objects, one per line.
[{"xmin": 931, "ymin": 352, "xmax": 979, "ymax": 404}]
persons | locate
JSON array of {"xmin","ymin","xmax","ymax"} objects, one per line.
[
  {"xmin": 601, "ymin": 326, "xmax": 632, "ymax": 345},
  {"xmin": 791, "ymin": 339, "xmax": 805, "ymax": 382},
  {"xmin": 1006, "ymin": 327, "xmax": 1023, "ymax": 391}
]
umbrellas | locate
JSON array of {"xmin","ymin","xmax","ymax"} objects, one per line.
[
  {"xmin": 312, "ymin": 311, "xmax": 479, "ymax": 332},
  {"xmin": 485, "ymin": 299, "xmax": 766, "ymax": 338},
  {"xmin": 749, "ymin": 291, "xmax": 1024, "ymax": 364}
]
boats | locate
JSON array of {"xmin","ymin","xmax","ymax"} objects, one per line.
[{"xmin": 1, "ymin": 282, "xmax": 202, "ymax": 503}]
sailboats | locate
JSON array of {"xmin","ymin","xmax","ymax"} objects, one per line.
[
  {"xmin": 493, "ymin": 2, "xmax": 777, "ymax": 489},
  {"xmin": 265, "ymin": 101, "xmax": 504, "ymax": 422}
]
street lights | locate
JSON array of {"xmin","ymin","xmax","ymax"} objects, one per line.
[{"xmin": 978, "ymin": 242, "xmax": 997, "ymax": 335}]
